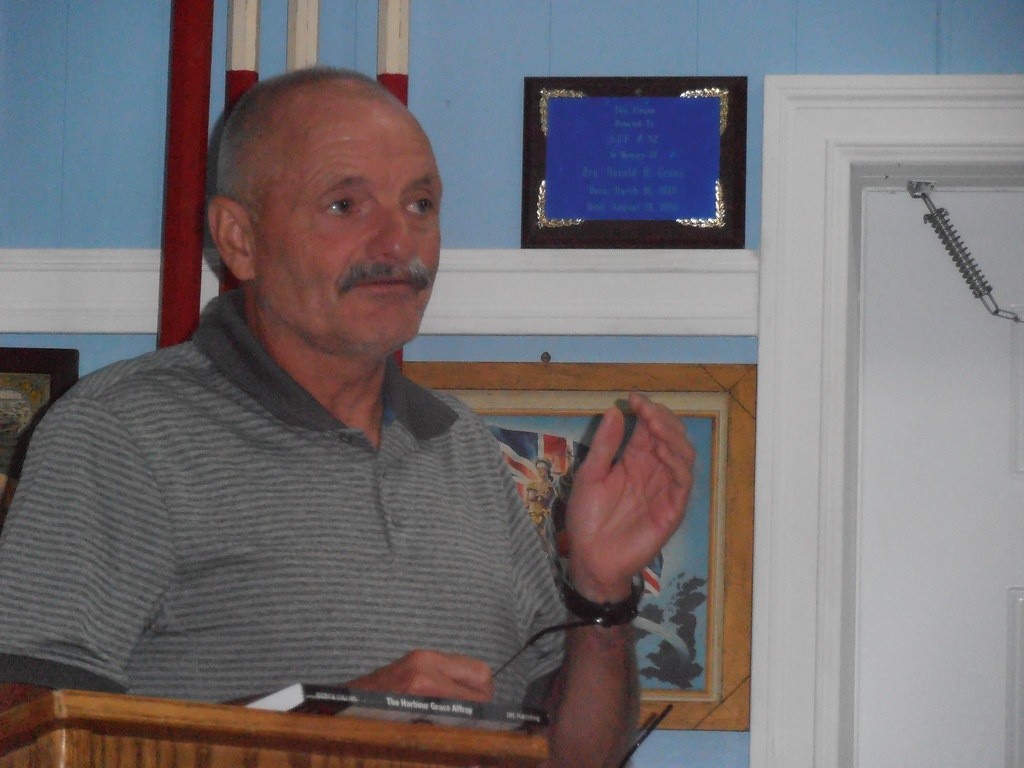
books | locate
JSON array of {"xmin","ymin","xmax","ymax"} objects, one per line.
[{"xmin": 245, "ymin": 683, "xmax": 551, "ymax": 733}]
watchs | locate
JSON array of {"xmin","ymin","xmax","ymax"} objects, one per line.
[{"xmin": 560, "ymin": 564, "xmax": 644, "ymax": 625}]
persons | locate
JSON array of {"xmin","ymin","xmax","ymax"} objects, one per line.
[{"xmin": 0, "ymin": 66, "xmax": 697, "ymax": 768}]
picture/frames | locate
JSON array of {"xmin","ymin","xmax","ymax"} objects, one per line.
[
  {"xmin": 518, "ymin": 75, "xmax": 748, "ymax": 250},
  {"xmin": 0, "ymin": 345, "xmax": 80, "ymax": 476},
  {"xmin": 403, "ymin": 360, "xmax": 758, "ymax": 728}
]
